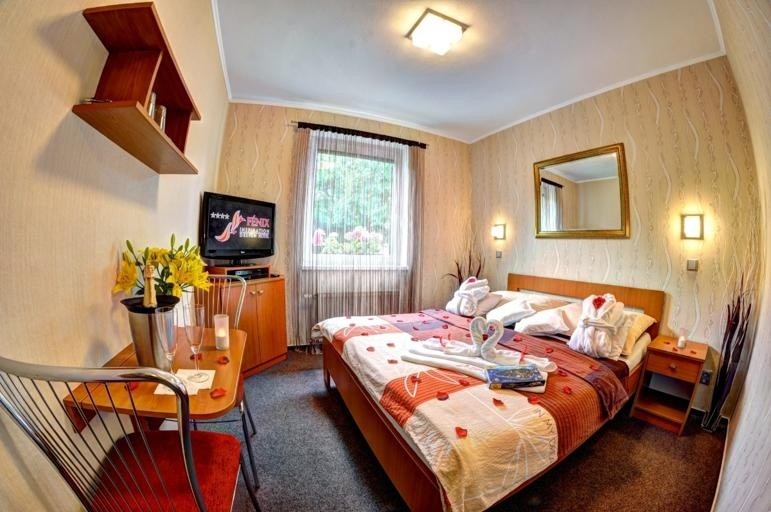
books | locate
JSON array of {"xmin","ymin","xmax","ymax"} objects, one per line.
[
  {"xmin": 149, "ymin": 92, "xmax": 157, "ymax": 121},
  {"xmin": 155, "ymin": 105, "xmax": 168, "ymax": 134}
]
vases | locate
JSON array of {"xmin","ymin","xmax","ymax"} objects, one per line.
[
  {"xmin": 700, "ymin": 355, "xmax": 741, "ymax": 433},
  {"xmin": 120, "ymin": 292, "xmax": 179, "ymax": 370}
]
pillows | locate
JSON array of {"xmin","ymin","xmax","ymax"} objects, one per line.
[
  {"xmin": 482, "ymin": 298, "xmax": 535, "ymax": 326},
  {"xmin": 557, "ymin": 301, "xmax": 581, "ymax": 333},
  {"xmin": 476, "ymin": 293, "xmax": 503, "ymax": 319},
  {"xmin": 492, "ymin": 291, "xmax": 570, "ymax": 313},
  {"xmin": 515, "ymin": 307, "xmax": 571, "ymax": 339},
  {"xmin": 620, "ymin": 309, "xmax": 658, "ymax": 355}
]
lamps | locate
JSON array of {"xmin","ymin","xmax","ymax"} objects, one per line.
[
  {"xmin": 680, "ymin": 213, "xmax": 705, "ymax": 240},
  {"xmin": 491, "ymin": 223, "xmax": 507, "ymax": 241},
  {"xmin": 401, "ymin": 6, "xmax": 472, "ymax": 59}
]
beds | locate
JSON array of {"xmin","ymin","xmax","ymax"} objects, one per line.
[{"xmin": 310, "ymin": 269, "xmax": 669, "ymax": 512}]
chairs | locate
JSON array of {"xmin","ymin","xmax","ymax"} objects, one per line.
[
  {"xmin": 177, "ymin": 271, "xmax": 262, "ymax": 491},
  {"xmin": 0, "ymin": 354, "xmax": 264, "ymax": 511}
]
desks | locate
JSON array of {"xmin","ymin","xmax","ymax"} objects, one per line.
[{"xmin": 61, "ymin": 322, "xmax": 250, "ymax": 441}]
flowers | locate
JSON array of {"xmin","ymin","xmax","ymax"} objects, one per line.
[{"xmin": 112, "ymin": 228, "xmax": 217, "ymax": 306}]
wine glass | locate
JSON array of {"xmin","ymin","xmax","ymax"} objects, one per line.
[
  {"xmin": 154, "ymin": 306, "xmax": 178, "ymax": 375},
  {"xmin": 182, "ymin": 303, "xmax": 212, "ymax": 385}
]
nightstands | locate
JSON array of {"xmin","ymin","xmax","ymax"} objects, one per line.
[{"xmin": 624, "ymin": 333, "xmax": 711, "ymax": 438}]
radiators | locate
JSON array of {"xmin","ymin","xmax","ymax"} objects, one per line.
[{"xmin": 304, "ymin": 288, "xmax": 407, "ymax": 353}]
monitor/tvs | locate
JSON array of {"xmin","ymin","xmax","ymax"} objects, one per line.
[{"xmin": 200, "ymin": 191, "xmax": 275, "ymax": 267}]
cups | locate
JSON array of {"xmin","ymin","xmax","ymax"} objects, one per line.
[{"xmin": 213, "ymin": 314, "xmax": 231, "ymax": 351}]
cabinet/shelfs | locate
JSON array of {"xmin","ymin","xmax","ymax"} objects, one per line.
[
  {"xmin": 69, "ymin": 0, "xmax": 202, "ymax": 176},
  {"xmin": 196, "ymin": 271, "xmax": 290, "ymax": 383}
]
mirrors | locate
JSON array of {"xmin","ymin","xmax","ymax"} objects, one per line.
[{"xmin": 533, "ymin": 142, "xmax": 631, "ymax": 240}]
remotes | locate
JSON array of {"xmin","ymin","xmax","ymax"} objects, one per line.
[{"xmin": 270, "ymin": 273, "xmax": 280, "ymax": 277}]
